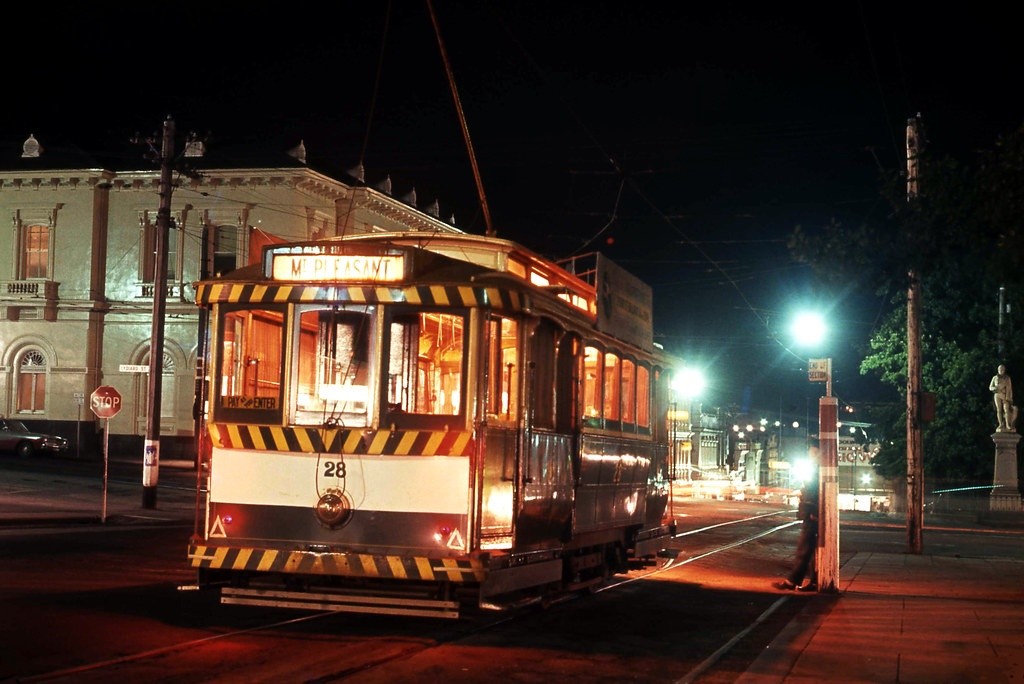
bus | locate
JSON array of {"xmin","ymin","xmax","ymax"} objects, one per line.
[{"xmin": 185, "ymin": 230, "xmax": 676, "ymax": 623}]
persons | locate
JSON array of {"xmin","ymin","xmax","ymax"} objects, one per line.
[
  {"xmin": 772, "ymin": 474, "xmax": 820, "ymax": 592},
  {"xmin": 989, "ymin": 365, "xmax": 1013, "ymax": 430}
]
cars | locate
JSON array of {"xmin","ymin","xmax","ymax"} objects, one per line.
[
  {"xmin": 674, "ymin": 473, "xmax": 803, "ymax": 506},
  {"xmin": 0, "ymin": 418, "xmax": 69, "ymax": 457}
]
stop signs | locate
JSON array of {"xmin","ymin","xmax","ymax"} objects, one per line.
[{"xmin": 90, "ymin": 385, "xmax": 123, "ymax": 419}]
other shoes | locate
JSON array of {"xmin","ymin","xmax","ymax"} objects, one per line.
[
  {"xmin": 772, "ymin": 581, "xmax": 795, "ymax": 590},
  {"xmin": 798, "ymin": 583, "xmax": 817, "ymax": 592}
]
